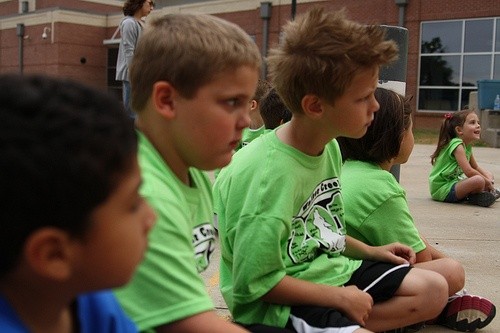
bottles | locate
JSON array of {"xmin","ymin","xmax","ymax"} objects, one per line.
[{"xmin": 494, "ymin": 95, "xmax": 500, "ymax": 110}]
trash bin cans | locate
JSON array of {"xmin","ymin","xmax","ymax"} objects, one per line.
[{"xmin": 477, "ymin": 80, "xmax": 500, "ymax": 110}]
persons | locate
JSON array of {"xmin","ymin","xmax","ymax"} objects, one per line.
[
  {"xmin": 339, "ymin": 88, "xmax": 496, "ymax": 332},
  {"xmin": 214, "ymin": 81, "xmax": 269, "ymax": 178},
  {"xmin": 429, "ymin": 111, "xmax": 500, "ymax": 207},
  {"xmin": 211, "ymin": 5, "xmax": 450, "ymax": 333},
  {"xmin": 260, "ymin": 86, "xmax": 293, "ymax": 134},
  {"xmin": 0, "ymin": 73, "xmax": 157, "ymax": 333},
  {"xmin": 115, "ymin": 0, "xmax": 156, "ymax": 120},
  {"xmin": 111, "ymin": 14, "xmax": 262, "ymax": 333}
]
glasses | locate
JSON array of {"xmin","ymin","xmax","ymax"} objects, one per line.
[{"xmin": 146, "ymin": 0, "xmax": 155, "ymax": 6}]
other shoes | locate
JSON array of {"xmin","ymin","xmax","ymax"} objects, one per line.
[
  {"xmin": 468, "ymin": 191, "xmax": 496, "ymax": 206},
  {"xmin": 490, "ymin": 187, "xmax": 500, "ymax": 199},
  {"xmin": 424, "ymin": 289, "xmax": 496, "ymax": 331}
]
